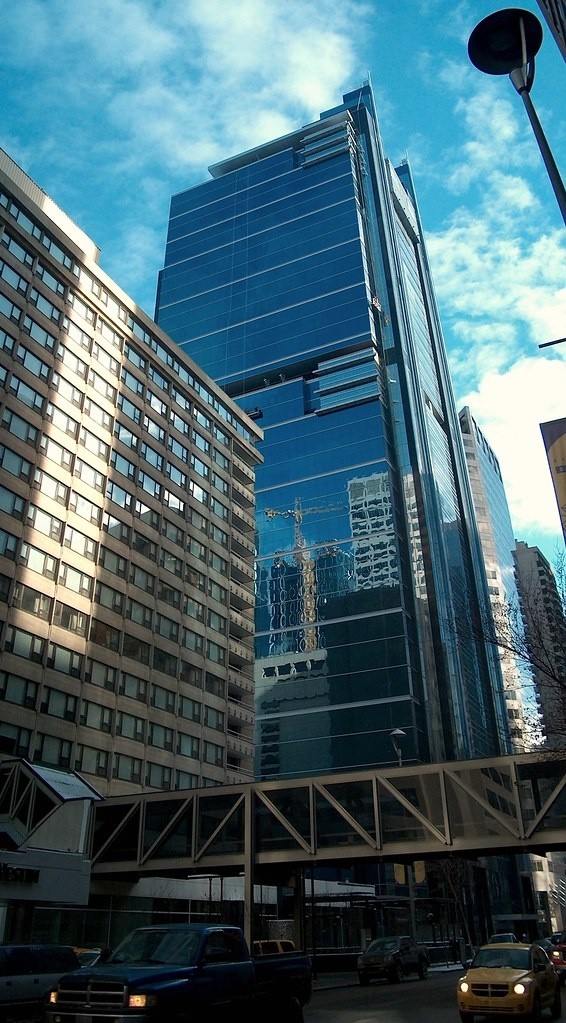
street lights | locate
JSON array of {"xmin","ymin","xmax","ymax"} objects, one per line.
[
  {"xmin": 467, "ymin": 8, "xmax": 566, "ymax": 226},
  {"xmin": 389, "ymin": 728, "xmax": 418, "ymax": 942}
]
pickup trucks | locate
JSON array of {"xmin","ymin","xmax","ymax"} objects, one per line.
[
  {"xmin": 44, "ymin": 923, "xmax": 312, "ymax": 1023},
  {"xmin": 0, "ymin": 945, "xmax": 81, "ymax": 1007}
]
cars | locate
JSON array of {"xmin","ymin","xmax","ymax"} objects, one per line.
[
  {"xmin": 356, "ymin": 936, "xmax": 431, "ymax": 984},
  {"xmin": 457, "ymin": 943, "xmax": 562, "ymax": 1023},
  {"xmin": 491, "ymin": 931, "xmax": 566, "ymax": 971}
]
